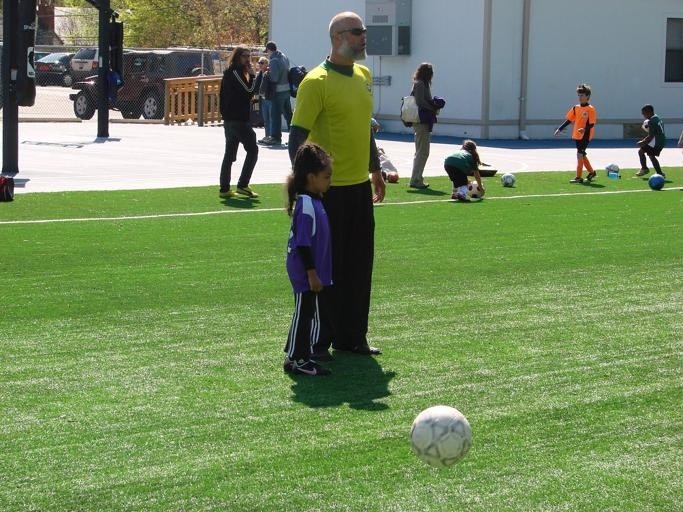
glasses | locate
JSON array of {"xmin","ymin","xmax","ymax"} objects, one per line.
[{"xmin": 338, "ymin": 28, "xmax": 367, "ymax": 35}]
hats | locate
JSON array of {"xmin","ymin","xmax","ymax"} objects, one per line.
[{"xmin": 263, "ymin": 42, "xmax": 276, "ymax": 53}]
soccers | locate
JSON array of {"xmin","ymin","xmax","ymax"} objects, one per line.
[
  {"xmin": 641, "ymin": 118, "xmax": 650, "ymax": 133},
  {"xmin": 649, "ymin": 174, "xmax": 664, "ymax": 190},
  {"xmin": 501, "ymin": 174, "xmax": 515, "ymax": 187},
  {"xmin": 605, "ymin": 164, "xmax": 619, "ymax": 176},
  {"xmin": 388, "ymin": 171, "xmax": 400, "ymax": 183},
  {"xmin": 411, "ymin": 405, "xmax": 472, "ymax": 466},
  {"xmin": 467, "ymin": 181, "xmax": 485, "ymax": 197}
]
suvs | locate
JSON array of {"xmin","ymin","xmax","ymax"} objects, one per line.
[
  {"xmin": 167, "ymin": 45, "xmax": 267, "ymax": 77},
  {"xmin": 70, "ymin": 47, "xmax": 111, "ymax": 84},
  {"xmin": 68, "ymin": 46, "xmax": 214, "ymax": 120}
]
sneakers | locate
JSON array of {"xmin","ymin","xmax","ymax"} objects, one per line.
[
  {"xmin": 411, "ymin": 184, "xmax": 430, "ymax": 188},
  {"xmin": 286, "ymin": 356, "xmax": 333, "ymax": 375},
  {"xmin": 635, "ymin": 166, "xmax": 649, "ymax": 176},
  {"xmin": 220, "ymin": 190, "xmax": 239, "ymax": 199},
  {"xmin": 451, "ymin": 191, "xmax": 467, "ymax": 199},
  {"xmin": 571, "ymin": 177, "xmax": 583, "ymax": 183},
  {"xmin": 313, "ymin": 349, "xmax": 336, "ymax": 363},
  {"xmin": 258, "ymin": 136, "xmax": 281, "ymax": 145},
  {"xmin": 237, "ymin": 188, "xmax": 258, "ymax": 199},
  {"xmin": 365, "ymin": 346, "xmax": 381, "ymax": 353},
  {"xmin": 585, "ymin": 171, "xmax": 596, "ymax": 181}
]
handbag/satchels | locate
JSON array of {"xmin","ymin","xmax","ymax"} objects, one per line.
[
  {"xmin": 401, "ymin": 96, "xmax": 433, "ymax": 123},
  {"xmin": 290, "ymin": 66, "xmax": 310, "ymax": 97}
]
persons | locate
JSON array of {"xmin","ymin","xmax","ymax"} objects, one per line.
[
  {"xmin": 288, "ymin": 12, "xmax": 386, "ymax": 353},
  {"xmin": 635, "ymin": 105, "xmax": 667, "ymax": 178},
  {"xmin": 217, "ymin": 44, "xmax": 261, "ymax": 198},
  {"xmin": 444, "ymin": 140, "xmax": 483, "ymax": 201},
  {"xmin": 258, "ymin": 41, "xmax": 307, "ymax": 146},
  {"xmin": 553, "ymin": 83, "xmax": 597, "ymax": 184},
  {"xmin": 283, "ymin": 142, "xmax": 334, "ymax": 374},
  {"xmin": 253, "ymin": 57, "xmax": 272, "ymax": 143},
  {"xmin": 410, "ymin": 63, "xmax": 446, "ymax": 188},
  {"xmin": 677, "ymin": 131, "xmax": 682, "ymax": 192}
]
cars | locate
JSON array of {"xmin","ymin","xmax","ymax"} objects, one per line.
[
  {"xmin": 33, "ymin": 51, "xmax": 49, "ymax": 62},
  {"xmin": 34, "ymin": 51, "xmax": 74, "ymax": 87}
]
